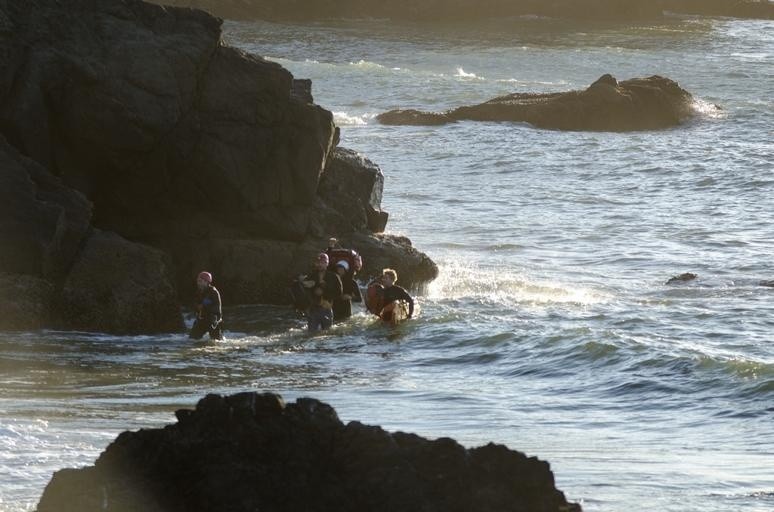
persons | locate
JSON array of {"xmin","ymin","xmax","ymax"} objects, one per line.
[
  {"xmin": 188, "ymin": 272, "xmax": 223, "ymax": 340},
  {"xmin": 291, "ymin": 238, "xmax": 413, "ymax": 330}
]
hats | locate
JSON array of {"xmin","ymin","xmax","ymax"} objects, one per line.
[
  {"xmin": 198, "ymin": 271, "xmax": 212, "ymax": 282},
  {"xmin": 336, "ymin": 261, "xmax": 349, "ymax": 270}
]
